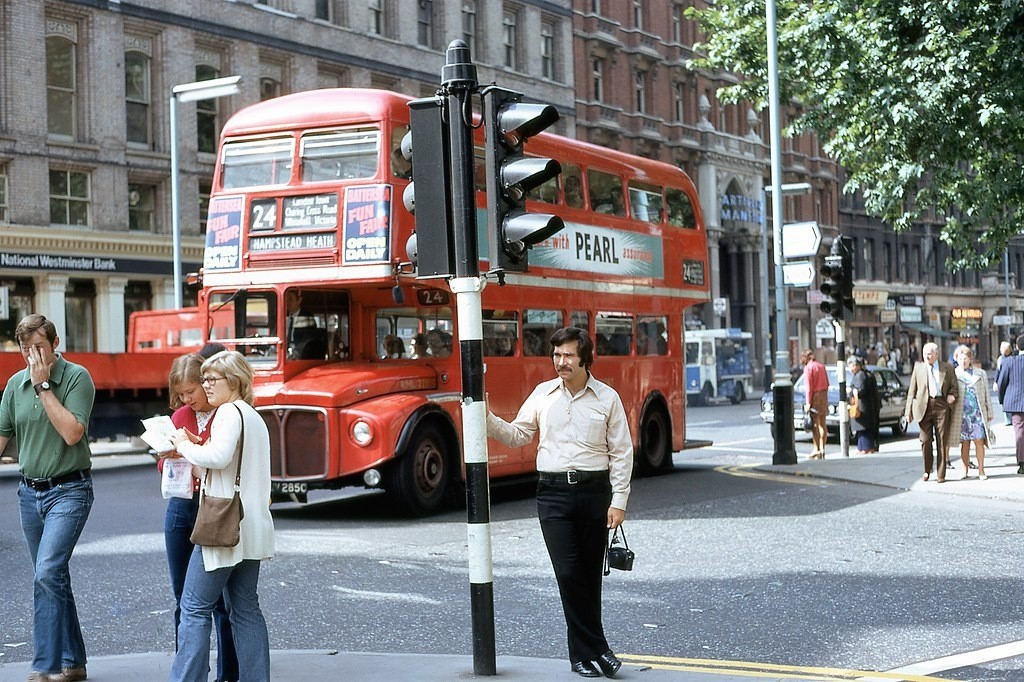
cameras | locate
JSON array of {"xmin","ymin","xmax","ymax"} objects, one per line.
[{"xmin": 609, "ymin": 547, "xmax": 634, "ymax": 571}]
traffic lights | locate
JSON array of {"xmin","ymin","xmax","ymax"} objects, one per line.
[
  {"xmin": 401, "ymin": 97, "xmax": 454, "ymax": 279},
  {"xmin": 820, "ymin": 257, "xmax": 845, "ymax": 322},
  {"xmin": 482, "ymin": 86, "xmax": 564, "ymax": 275}
]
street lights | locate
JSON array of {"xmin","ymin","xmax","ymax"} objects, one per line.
[{"xmin": 170, "ymin": 74, "xmax": 242, "ymax": 310}]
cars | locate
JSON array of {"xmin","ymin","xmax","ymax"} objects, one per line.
[{"xmin": 761, "ymin": 365, "xmax": 911, "ymax": 444}]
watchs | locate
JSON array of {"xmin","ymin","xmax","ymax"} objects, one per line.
[
  {"xmin": 34, "ymin": 381, "xmax": 50, "ymax": 396},
  {"xmin": 196, "ymin": 436, "xmax": 202, "ymax": 441}
]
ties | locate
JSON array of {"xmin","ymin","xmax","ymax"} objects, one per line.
[{"xmin": 929, "ymin": 364, "xmax": 937, "ymax": 398}]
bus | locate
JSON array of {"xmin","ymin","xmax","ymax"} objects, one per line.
[{"xmin": 198, "ymin": 88, "xmax": 713, "ymax": 521}]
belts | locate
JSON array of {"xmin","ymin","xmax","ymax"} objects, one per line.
[
  {"xmin": 540, "ymin": 468, "xmax": 610, "ymax": 484},
  {"xmin": 20, "ymin": 467, "xmax": 92, "ymax": 492}
]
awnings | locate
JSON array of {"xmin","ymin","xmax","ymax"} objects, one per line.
[{"xmin": 902, "ymin": 323, "xmax": 956, "ymax": 337}]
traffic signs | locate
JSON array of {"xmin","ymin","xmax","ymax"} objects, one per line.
[
  {"xmin": 781, "ymin": 222, "xmax": 820, "ymax": 258},
  {"xmin": 783, "ymin": 261, "xmax": 816, "ymax": 288}
]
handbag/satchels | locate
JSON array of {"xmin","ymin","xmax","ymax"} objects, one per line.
[
  {"xmin": 850, "ymin": 406, "xmax": 860, "ymax": 418},
  {"xmin": 607, "ymin": 547, "xmax": 634, "ymax": 571},
  {"xmin": 988, "ymin": 429, "xmax": 996, "ymax": 444},
  {"xmin": 804, "ymin": 415, "xmax": 813, "ymax": 433},
  {"xmin": 190, "ymin": 492, "xmax": 245, "ymax": 547}
]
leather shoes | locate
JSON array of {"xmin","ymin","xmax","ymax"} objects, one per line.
[
  {"xmin": 596, "ymin": 648, "xmax": 622, "ymax": 678},
  {"xmin": 571, "ymin": 659, "xmax": 600, "ymax": 677}
]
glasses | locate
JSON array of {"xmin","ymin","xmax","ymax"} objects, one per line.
[{"xmin": 199, "ymin": 375, "xmax": 231, "ymax": 386}]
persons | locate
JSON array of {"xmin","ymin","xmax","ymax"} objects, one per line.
[
  {"xmin": 611, "ymin": 186, "xmax": 626, "ymax": 216},
  {"xmin": 800, "ymin": 348, "xmax": 829, "ymax": 459},
  {"xmin": 597, "ymin": 320, "xmax": 667, "ymax": 355},
  {"xmin": 947, "ymin": 345, "xmax": 993, "ymax": 481},
  {"xmin": 0, "ymin": 315, "xmax": 96, "ymax": 682},
  {"xmin": 382, "ymin": 335, "xmax": 400, "ymax": 359},
  {"xmin": 409, "ymin": 330, "xmax": 452, "ymax": 360},
  {"xmin": 652, "ymin": 200, "xmax": 684, "ymax": 226},
  {"xmin": 523, "ymin": 332, "xmax": 538, "ymax": 356},
  {"xmin": 848, "ymin": 342, "xmax": 917, "ymax": 376},
  {"xmin": 483, "ymin": 329, "xmax": 514, "ymax": 357},
  {"xmin": 847, "ymin": 356, "xmax": 882, "ymax": 454},
  {"xmin": 565, "ymin": 175, "xmax": 582, "ymax": 208},
  {"xmin": 905, "ymin": 343, "xmax": 959, "ymax": 483},
  {"xmin": 266, "ymin": 290, "xmax": 327, "ymax": 360},
  {"xmin": 993, "ymin": 334, "xmax": 1024, "ymax": 474},
  {"xmin": 461, "ymin": 326, "xmax": 634, "ymax": 678},
  {"xmin": 156, "ymin": 344, "xmax": 276, "ymax": 682}
]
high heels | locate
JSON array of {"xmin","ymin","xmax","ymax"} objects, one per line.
[{"xmin": 809, "ymin": 449, "xmax": 825, "ymax": 461}]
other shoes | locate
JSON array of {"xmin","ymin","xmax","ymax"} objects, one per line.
[
  {"xmin": 979, "ymin": 473, "xmax": 987, "ymax": 480},
  {"xmin": 923, "ymin": 472, "xmax": 930, "ymax": 481},
  {"xmin": 946, "ymin": 461, "xmax": 954, "ymax": 469},
  {"xmin": 49, "ymin": 665, "xmax": 87, "ymax": 682},
  {"xmin": 937, "ymin": 478, "xmax": 945, "ymax": 483},
  {"xmin": 857, "ymin": 449, "xmax": 875, "ymax": 455},
  {"xmin": 1017, "ymin": 466, "xmax": 1024, "ymax": 475},
  {"xmin": 969, "ymin": 461, "xmax": 976, "ymax": 469},
  {"xmin": 26, "ymin": 671, "xmax": 48, "ymax": 682}
]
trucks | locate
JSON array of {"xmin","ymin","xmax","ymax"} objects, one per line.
[
  {"xmin": 659, "ymin": 328, "xmax": 753, "ymax": 407},
  {"xmin": 0, "ymin": 303, "xmax": 270, "ymax": 438}
]
